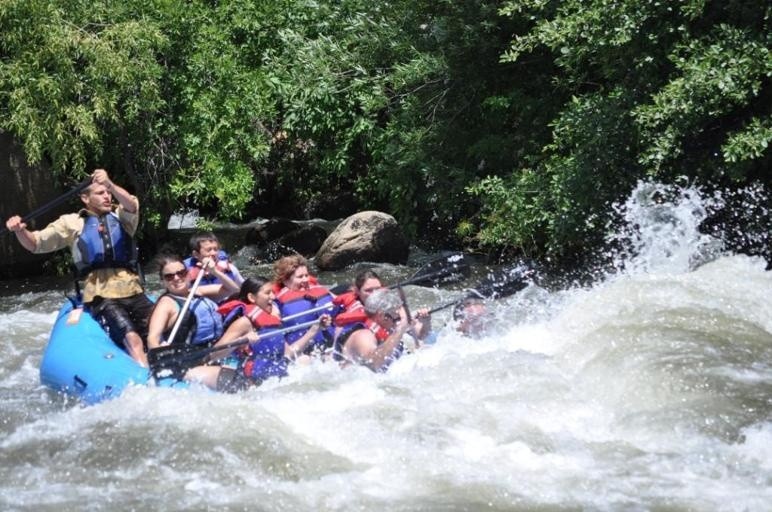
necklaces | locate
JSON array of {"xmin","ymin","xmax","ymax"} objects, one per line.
[{"xmin": 333, "ymin": 289, "xmax": 416, "ymax": 376}]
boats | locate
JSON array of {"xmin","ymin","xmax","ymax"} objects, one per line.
[{"xmin": 34, "ymin": 281, "xmax": 439, "ymax": 411}]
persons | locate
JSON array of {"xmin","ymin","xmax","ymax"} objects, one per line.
[
  {"xmin": 182, "ymin": 231, "xmax": 247, "ymax": 304},
  {"xmin": 5, "ymin": 167, "xmax": 157, "ymax": 367},
  {"xmin": 450, "ymin": 298, "xmax": 489, "ymax": 340},
  {"xmin": 145, "ymin": 255, "xmax": 249, "ymax": 395},
  {"xmin": 269, "ymin": 255, "xmax": 344, "ymax": 354},
  {"xmin": 209, "ymin": 275, "xmax": 332, "ymax": 388},
  {"xmin": 333, "ymin": 270, "xmax": 433, "ymax": 341}
]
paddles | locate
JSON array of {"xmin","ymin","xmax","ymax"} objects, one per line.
[
  {"xmin": 413, "ymin": 257, "xmax": 536, "ymax": 318},
  {"xmin": 280, "ymin": 250, "xmax": 471, "ymax": 330},
  {"xmin": 147, "ymin": 320, "xmax": 324, "ymax": 381}
]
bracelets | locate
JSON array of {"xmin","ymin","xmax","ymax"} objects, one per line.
[{"xmin": 107, "ymin": 180, "xmax": 113, "ymax": 191}]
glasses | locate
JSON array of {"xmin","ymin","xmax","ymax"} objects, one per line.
[
  {"xmin": 165, "ymin": 270, "xmax": 187, "ymax": 280},
  {"xmin": 385, "ymin": 313, "xmax": 401, "ymax": 324}
]
